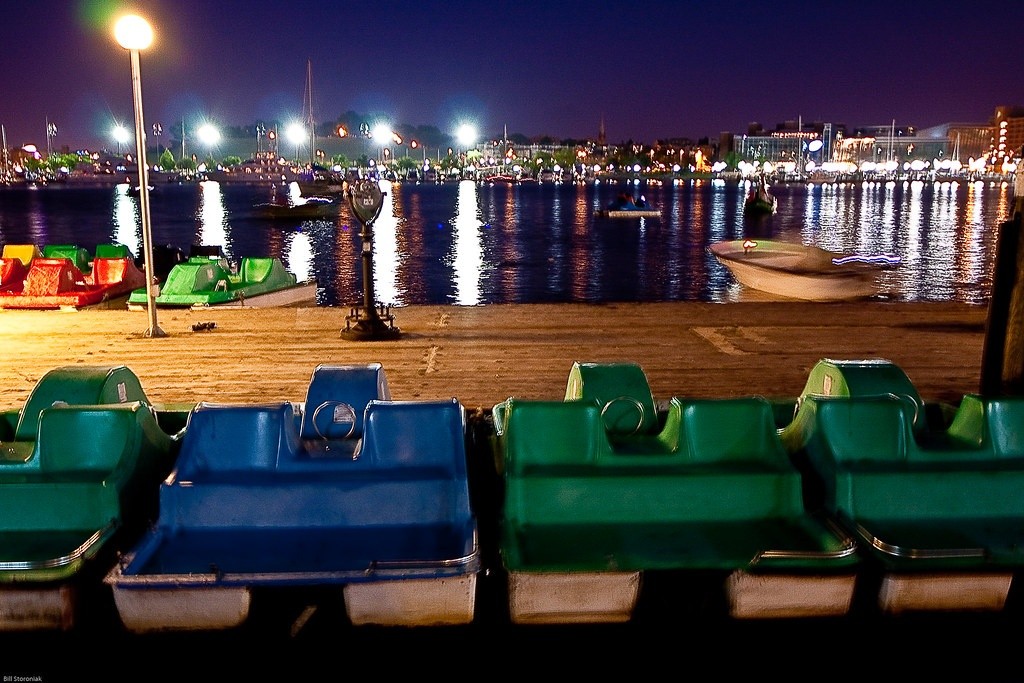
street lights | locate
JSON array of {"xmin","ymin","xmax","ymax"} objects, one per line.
[{"xmin": 113, "ymin": 14, "xmax": 170, "ymax": 339}]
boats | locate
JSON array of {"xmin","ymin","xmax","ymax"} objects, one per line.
[
  {"xmin": 100, "ymin": 361, "xmax": 496, "ymax": 628},
  {"xmin": 708, "ymin": 236, "xmax": 882, "ymax": 305},
  {"xmin": 593, "ymin": 196, "xmax": 662, "ymax": 218},
  {"xmin": 743, "ymin": 182, "xmax": 779, "ymax": 216},
  {"xmin": 129, "ymin": 255, "xmax": 299, "ymax": 306},
  {"xmin": 789, "ymin": 357, "xmax": 1024, "ymax": 615},
  {"xmin": 486, "ymin": 362, "xmax": 859, "ymax": 631},
  {"xmin": 0, "ymin": 242, "xmax": 160, "ymax": 309},
  {"xmin": 0, "ymin": 366, "xmax": 197, "ymax": 630}
]
